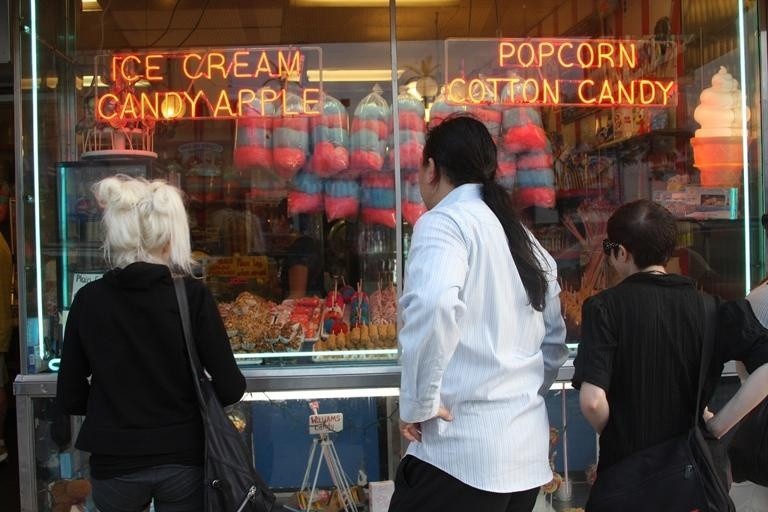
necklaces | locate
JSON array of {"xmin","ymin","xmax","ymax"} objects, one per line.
[{"xmin": 647, "ymin": 269, "xmax": 666, "ymax": 275}]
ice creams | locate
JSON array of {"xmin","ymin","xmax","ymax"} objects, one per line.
[{"xmin": 689, "ymin": 63, "xmax": 753, "ymax": 188}]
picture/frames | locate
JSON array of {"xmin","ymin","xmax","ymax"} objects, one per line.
[{"xmin": 557, "ymin": 9, "xmax": 614, "ymax": 125}]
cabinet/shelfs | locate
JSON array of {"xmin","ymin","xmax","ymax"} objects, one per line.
[{"xmin": 536, "ymin": 186, "xmax": 617, "ymax": 264}]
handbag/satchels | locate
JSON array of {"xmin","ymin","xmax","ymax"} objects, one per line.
[
  {"xmin": 586, "ymin": 429, "xmax": 735, "ymax": 512},
  {"xmin": 203, "ymin": 376, "xmax": 275, "ymax": 511}
]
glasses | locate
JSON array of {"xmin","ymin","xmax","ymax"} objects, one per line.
[{"xmin": 604, "ymin": 238, "xmax": 622, "ymax": 255}]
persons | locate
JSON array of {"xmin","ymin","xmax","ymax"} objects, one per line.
[
  {"xmin": 567, "ymin": 198, "xmax": 768, "ymax": 511},
  {"xmin": 380, "ymin": 111, "xmax": 574, "ymax": 511},
  {"xmin": 54, "ymin": 169, "xmax": 247, "ymax": 511},
  {"xmin": 266, "ymin": 196, "xmax": 328, "ymax": 303},
  {"xmin": 0, "ymin": 230, "xmax": 16, "ymax": 463},
  {"xmin": 724, "ymin": 214, "xmax": 768, "ymax": 512}
]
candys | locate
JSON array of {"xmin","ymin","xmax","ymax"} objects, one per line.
[
  {"xmin": 547, "ymin": 423, "xmax": 559, "ymax": 466},
  {"xmin": 540, "ymin": 472, "xmax": 562, "ymax": 512}
]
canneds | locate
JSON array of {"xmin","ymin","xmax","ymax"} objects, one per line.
[{"xmin": 25, "ymin": 315, "xmax": 54, "ymax": 375}]
